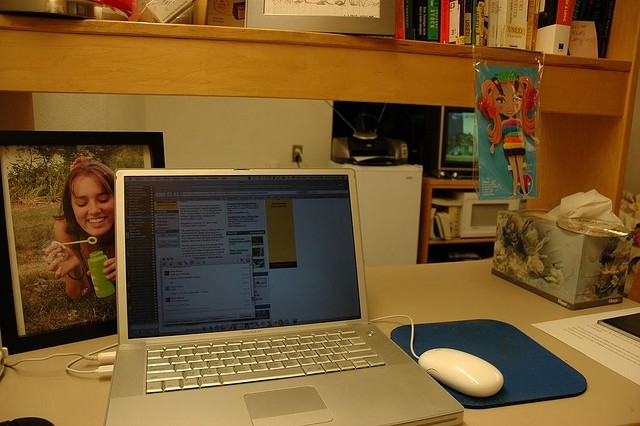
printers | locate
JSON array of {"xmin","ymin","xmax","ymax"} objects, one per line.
[{"xmin": 332, "ymin": 131, "xmax": 409, "ymax": 167}]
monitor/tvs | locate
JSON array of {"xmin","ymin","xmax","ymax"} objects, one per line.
[{"xmin": 431, "ymin": 106, "xmax": 479, "ymax": 180}]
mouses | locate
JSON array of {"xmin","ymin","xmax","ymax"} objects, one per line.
[{"xmin": 417, "ymin": 346, "xmax": 505, "ymax": 400}]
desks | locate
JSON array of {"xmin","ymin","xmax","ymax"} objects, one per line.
[{"xmin": 0, "ymin": 258, "xmax": 639, "ymax": 424}]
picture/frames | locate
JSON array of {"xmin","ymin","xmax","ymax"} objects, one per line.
[
  {"xmin": 0, "ymin": 130, "xmax": 165, "ymax": 356},
  {"xmin": 245, "ymin": 1, "xmax": 396, "ymax": 39}
]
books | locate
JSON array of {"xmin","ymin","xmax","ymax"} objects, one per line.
[
  {"xmin": 395, "ymin": 1, "xmax": 616, "ymax": 58},
  {"xmin": 598, "ymin": 313, "xmax": 640, "ymax": 342}
]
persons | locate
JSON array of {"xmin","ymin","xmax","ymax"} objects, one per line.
[{"xmin": 45, "ymin": 162, "xmax": 115, "ymax": 301}]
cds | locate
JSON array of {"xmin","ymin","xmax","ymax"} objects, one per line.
[{"xmin": 558, "ymin": 217, "xmax": 633, "ymax": 240}]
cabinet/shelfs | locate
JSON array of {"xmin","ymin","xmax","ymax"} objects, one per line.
[{"xmin": 0, "ymin": 1, "xmax": 639, "ymax": 263}]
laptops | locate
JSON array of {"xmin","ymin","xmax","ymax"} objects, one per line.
[{"xmin": 103, "ymin": 168, "xmax": 466, "ymax": 425}]
